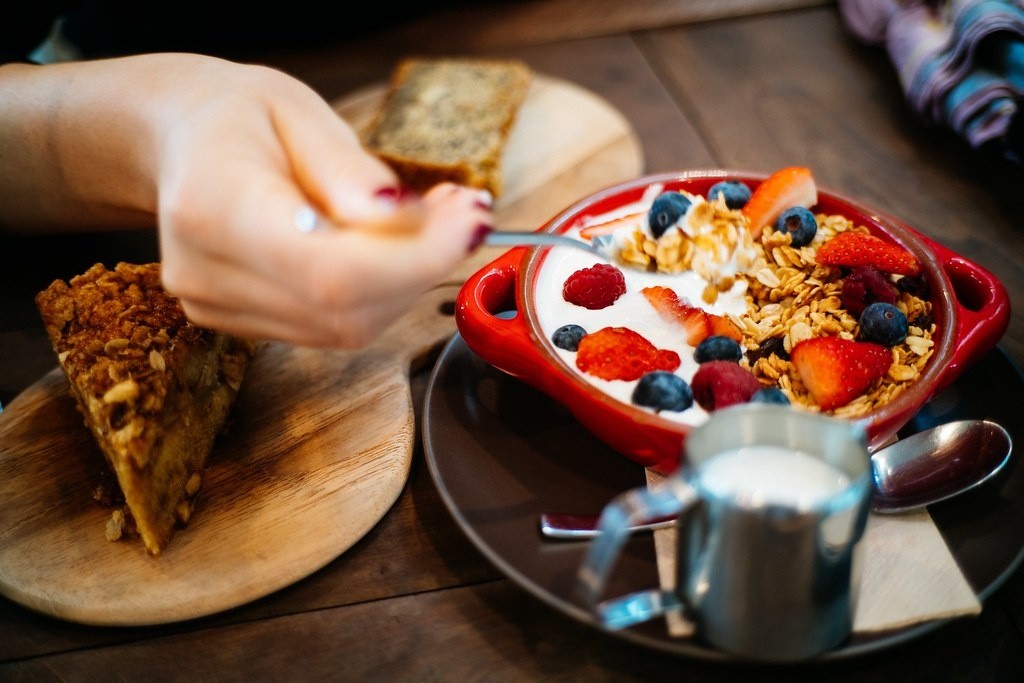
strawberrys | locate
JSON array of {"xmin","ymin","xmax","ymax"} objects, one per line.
[
  {"xmin": 576, "ymin": 326, "xmax": 683, "ymax": 383},
  {"xmin": 740, "ymin": 166, "xmax": 921, "ymax": 275},
  {"xmin": 788, "ymin": 337, "xmax": 895, "ymax": 409}
]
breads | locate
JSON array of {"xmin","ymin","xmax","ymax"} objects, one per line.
[{"xmin": 353, "ymin": 58, "xmax": 532, "ymax": 197}]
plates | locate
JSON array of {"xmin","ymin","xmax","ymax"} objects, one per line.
[{"xmin": 421, "ymin": 311, "xmax": 1024, "ymax": 665}]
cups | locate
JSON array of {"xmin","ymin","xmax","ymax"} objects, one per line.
[{"xmin": 573, "ymin": 403, "xmax": 877, "ymax": 665}]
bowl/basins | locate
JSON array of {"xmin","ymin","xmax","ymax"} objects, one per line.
[{"xmin": 454, "ymin": 168, "xmax": 1012, "ymax": 479}]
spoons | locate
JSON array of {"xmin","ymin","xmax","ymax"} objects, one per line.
[
  {"xmin": 295, "ymin": 204, "xmax": 693, "ymax": 275},
  {"xmin": 539, "ymin": 417, "xmax": 1014, "ymax": 542}
]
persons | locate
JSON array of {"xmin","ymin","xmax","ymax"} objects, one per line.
[{"xmin": 0, "ymin": 1, "xmax": 542, "ymax": 352}]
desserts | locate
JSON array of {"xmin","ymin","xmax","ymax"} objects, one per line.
[{"xmin": 36, "ymin": 261, "xmax": 259, "ymax": 558}]
